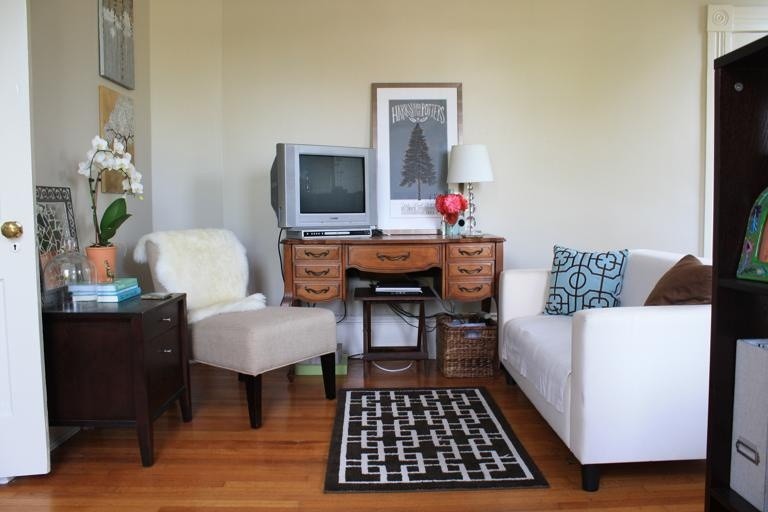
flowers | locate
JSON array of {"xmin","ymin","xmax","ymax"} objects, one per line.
[
  {"xmin": 435, "ymin": 194, "xmax": 468, "ymax": 225},
  {"xmin": 77, "ymin": 129, "xmax": 144, "ymax": 247}
]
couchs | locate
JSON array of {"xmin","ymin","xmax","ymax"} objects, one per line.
[{"xmin": 498, "ymin": 249, "xmax": 712, "ymax": 492}]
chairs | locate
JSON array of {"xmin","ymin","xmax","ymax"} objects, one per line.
[{"xmin": 132, "ymin": 229, "xmax": 337, "ymax": 429}]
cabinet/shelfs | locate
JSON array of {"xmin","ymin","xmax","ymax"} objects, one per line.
[
  {"xmin": 42, "ymin": 293, "xmax": 193, "ymax": 467},
  {"xmin": 703, "ymin": 37, "xmax": 768, "ymax": 510}
]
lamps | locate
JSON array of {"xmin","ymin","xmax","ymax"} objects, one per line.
[{"xmin": 445, "ymin": 145, "xmax": 494, "ymax": 237}]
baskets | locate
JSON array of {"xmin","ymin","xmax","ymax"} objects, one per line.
[{"xmin": 436, "ymin": 313, "xmax": 499, "ymax": 378}]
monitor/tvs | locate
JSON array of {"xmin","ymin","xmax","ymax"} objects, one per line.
[{"xmin": 270, "ymin": 143, "xmax": 379, "ymax": 232}]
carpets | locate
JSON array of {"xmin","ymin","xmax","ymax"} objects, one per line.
[{"xmin": 323, "ymin": 387, "xmax": 550, "ymax": 494}]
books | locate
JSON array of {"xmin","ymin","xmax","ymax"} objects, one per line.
[{"xmin": 68, "ymin": 278, "xmax": 142, "ymax": 303}]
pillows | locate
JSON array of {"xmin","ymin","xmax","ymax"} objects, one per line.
[
  {"xmin": 540, "ymin": 244, "xmax": 630, "ymax": 316},
  {"xmin": 644, "ymin": 254, "xmax": 712, "ymax": 306}
]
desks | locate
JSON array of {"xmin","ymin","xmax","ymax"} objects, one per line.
[{"xmin": 279, "ymin": 235, "xmax": 507, "ymax": 383}]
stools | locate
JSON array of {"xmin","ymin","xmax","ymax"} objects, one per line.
[{"xmin": 354, "ymin": 287, "xmax": 437, "ymax": 379}]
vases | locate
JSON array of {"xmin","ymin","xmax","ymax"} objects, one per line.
[
  {"xmin": 86, "ymin": 246, "xmax": 116, "ymax": 282},
  {"xmin": 445, "ymin": 218, "xmax": 465, "ymax": 236}
]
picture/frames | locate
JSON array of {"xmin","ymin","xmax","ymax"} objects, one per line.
[
  {"xmin": 370, "ymin": 83, "xmax": 463, "ymax": 235},
  {"xmin": 34, "ymin": 186, "xmax": 84, "ymax": 308}
]
